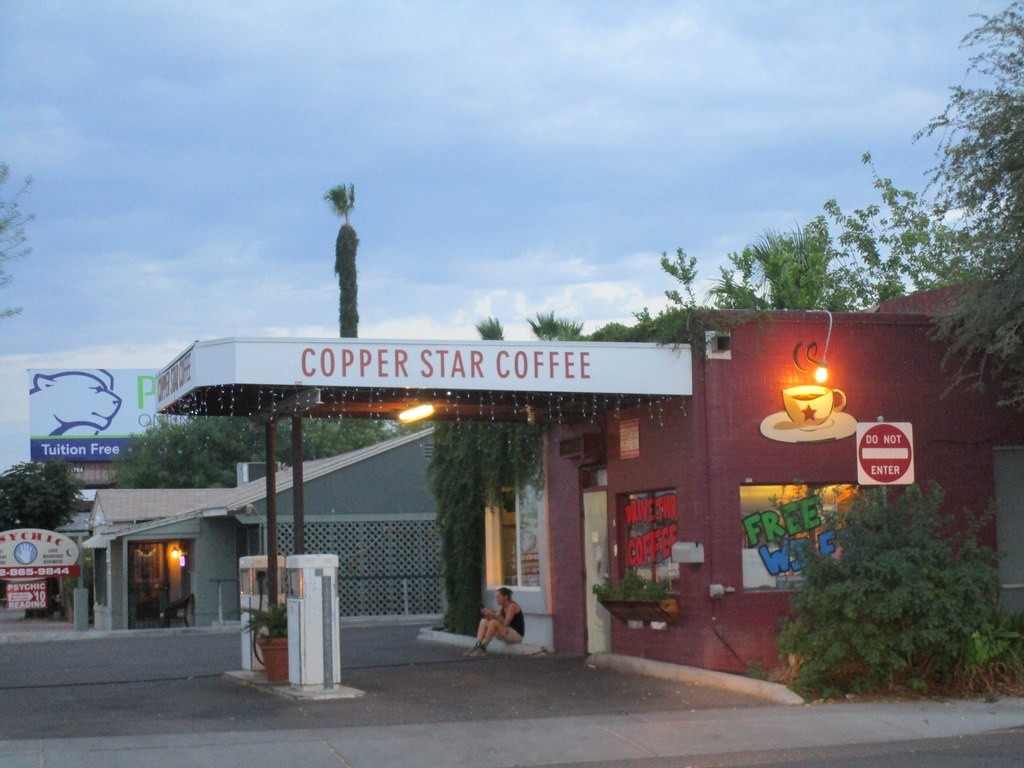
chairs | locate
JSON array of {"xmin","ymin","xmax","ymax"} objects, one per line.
[
  {"xmin": 160, "ymin": 592, "xmax": 194, "ymax": 629},
  {"xmin": 135, "ymin": 599, "xmax": 163, "ymax": 629}
]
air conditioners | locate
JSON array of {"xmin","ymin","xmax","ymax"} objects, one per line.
[
  {"xmin": 237, "ymin": 461, "xmax": 282, "ymax": 488},
  {"xmin": 558, "ymin": 433, "xmax": 603, "ymax": 466}
]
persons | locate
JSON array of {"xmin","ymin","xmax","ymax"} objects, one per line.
[{"xmin": 464, "ymin": 588, "xmax": 525, "ymax": 658}]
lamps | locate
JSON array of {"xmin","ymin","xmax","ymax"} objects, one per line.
[
  {"xmin": 171, "ymin": 542, "xmax": 181, "ymax": 559},
  {"xmin": 808, "ymin": 309, "xmax": 833, "ymax": 383},
  {"xmin": 515, "ymin": 405, "xmax": 535, "ymax": 424}
]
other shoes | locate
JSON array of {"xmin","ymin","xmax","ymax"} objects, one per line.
[{"xmin": 462, "ymin": 646, "xmax": 487, "ymax": 657}]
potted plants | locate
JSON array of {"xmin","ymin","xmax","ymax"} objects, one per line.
[
  {"xmin": 225, "ymin": 599, "xmax": 288, "ymax": 683},
  {"xmin": 590, "ymin": 568, "xmax": 678, "ymax": 626}
]
